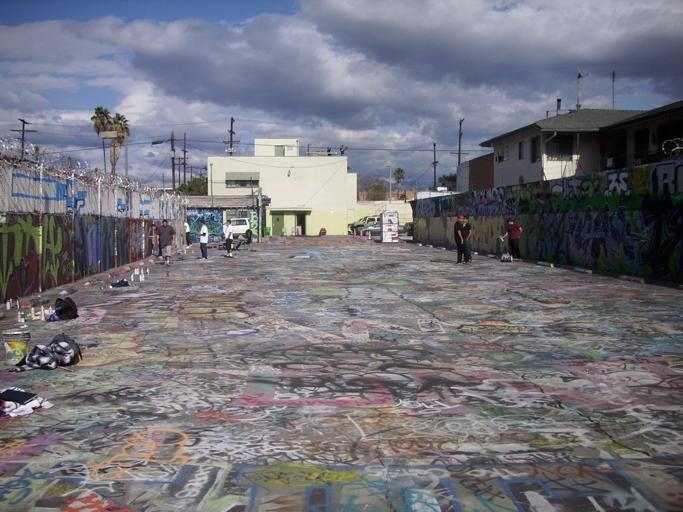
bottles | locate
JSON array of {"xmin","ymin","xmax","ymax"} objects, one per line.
[
  {"xmin": 5, "ymin": 297, "xmax": 53, "ymax": 322},
  {"xmin": 129, "ymin": 267, "xmax": 150, "ymax": 283}
]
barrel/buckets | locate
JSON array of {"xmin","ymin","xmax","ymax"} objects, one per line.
[{"xmin": 2, "ymin": 329, "xmax": 31, "ymax": 366}]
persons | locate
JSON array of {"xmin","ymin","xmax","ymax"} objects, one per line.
[
  {"xmin": 148, "ymin": 220, "xmax": 176, "ymax": 266},
  {"xmin": 455, "ymin": 214, "xmax": 472, "ymax": 265},
  {"xmin": 183, "ymin": 220, "xmax": 192, "ymax": 248},
  {"xmin": 502, "ymin": 217, "xmax": 522, "ymax": 259},
  {"xmin": 225, "ymin": 220, "xmax": 234, "ymax": 257},
  {"xmin": 199, "ymin": 220, "xmax": 208, "ymax": 260}
]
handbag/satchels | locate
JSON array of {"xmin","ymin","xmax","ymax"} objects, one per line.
[{"xmin": 54, "ymin": 297, "xmax": 78, "ymax": 320}]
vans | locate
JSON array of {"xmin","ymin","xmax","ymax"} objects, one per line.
[{"xmin": 222, "ymin": 218, "xmax": 251, "ymax": 237}]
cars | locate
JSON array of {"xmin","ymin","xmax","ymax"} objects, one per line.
[{"xmin": 348, "ymin": 215, "xmax": 414, "ymax": 236}]
